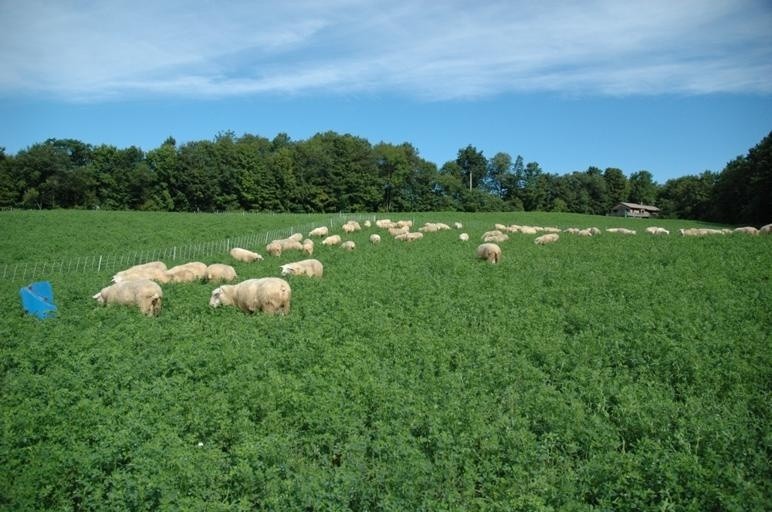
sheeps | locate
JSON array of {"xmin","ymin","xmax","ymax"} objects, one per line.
[
  {"xmin": 341, "ymin": 219, "xmax": 470, "ymax": 243},
  {"xmin": 265, "ymin": 226, "xmax": 355, "ymax": 255},
  {"xmin": 93, "ymin": 278, "xmax": 163, "ymax": 317},
  {"xmin": 230, "ymin": 247, "xmax": 264, "ymax": 264},
  {"xmin": 209, "ymin": 277, "xmax": 292, "ymax": 317},
  {"xmin": 475, "ymin": 243, "xmax": 501, "ymax": 265},
  {"xmin": 480, "ymin": 223, "xmax": 771, "ymax": 245},
  {"xmin": 112, "ymin": 260, "xmax": 237, "ymax": 283},
  {"xmin": 281, "ymin": 259, "xmax": 324, "ymax": 278}
]
